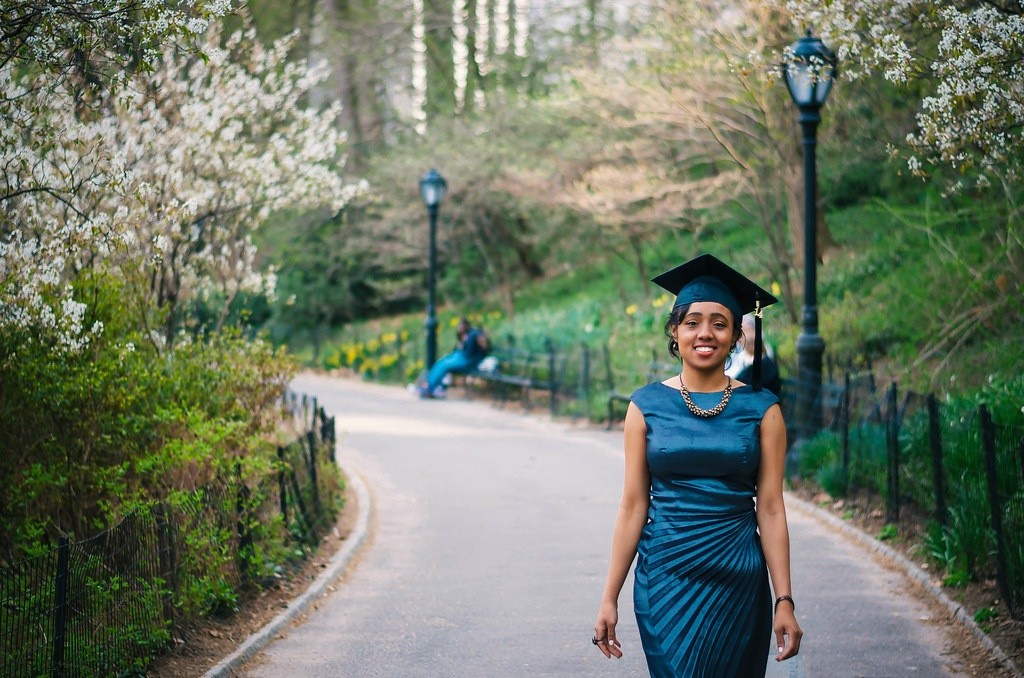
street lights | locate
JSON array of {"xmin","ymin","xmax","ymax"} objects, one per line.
[
  {"xmin": 416, "ymin": 166, "xmax": 447, "ymax": 373},
  {"xmin": 780, "ymin": 27, "xmax": 839, "ymax": 446}
]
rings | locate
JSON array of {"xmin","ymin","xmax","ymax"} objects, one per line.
[{"xmin": 592, "ymin": 636, "xmax": 604, "ymax": 645}]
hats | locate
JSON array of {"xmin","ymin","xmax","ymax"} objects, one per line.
[{"xmin": 650, "ymin": 253, "xmax": 779, "ymax": 392}]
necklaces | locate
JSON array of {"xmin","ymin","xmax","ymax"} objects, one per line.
[{"xmin": 679, "ymin": 373, "xmax": 733, "ymax": 417}]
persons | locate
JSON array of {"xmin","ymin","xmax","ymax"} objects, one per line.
[
  {"xmin": 408, "ymin": 320, "xmax": 491, "ymax": 399},
  {"xmin": 724, "ymin": 312, "xmax": 782, "ymax": 398},
  {"xmin": 591, "ymin": 254, "xmax": 803, "ymax": 678}
]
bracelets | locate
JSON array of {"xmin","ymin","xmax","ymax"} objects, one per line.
[{"xmin": 775, "ymin": 595, "xmax": 794, "ymax": 614}]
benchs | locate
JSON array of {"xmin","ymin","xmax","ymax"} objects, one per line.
[
  {"xmin": 605, "ymin": 362, "xmax": 683, "ymax": 430},
  {"xmin": 453, "ymin": 342, "xmax": 567, "ymax": 409}
]
